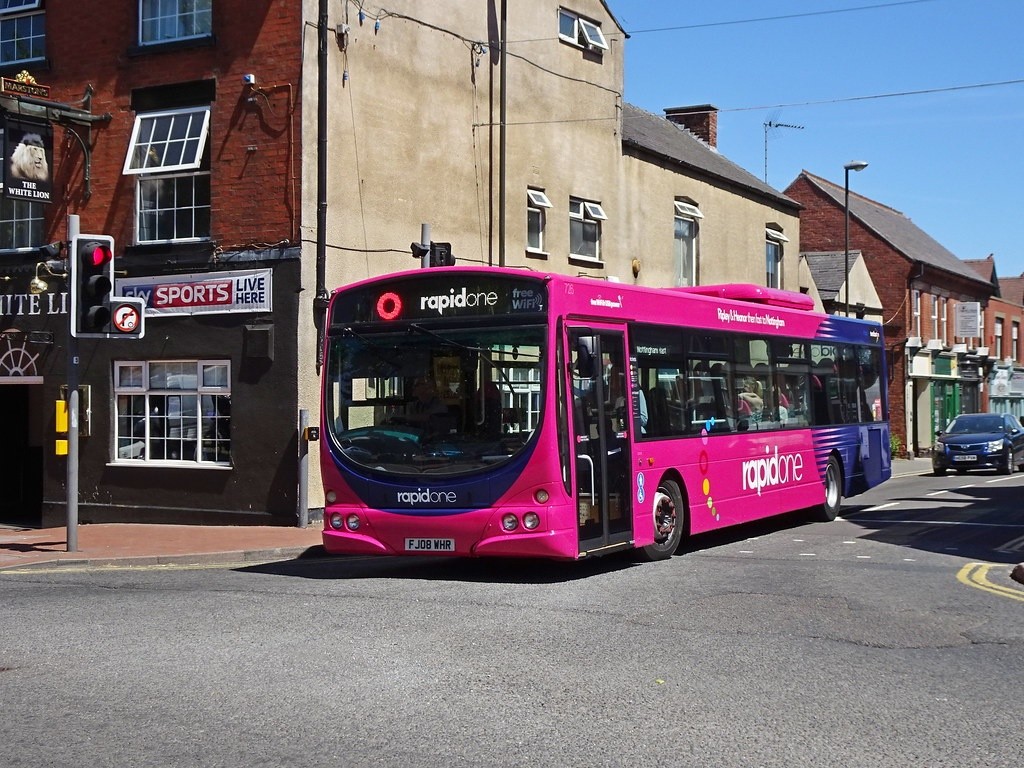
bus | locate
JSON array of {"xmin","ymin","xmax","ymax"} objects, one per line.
[{"xmin": 319, "ymin": 265, "xmax": 891, "ymax": 564}]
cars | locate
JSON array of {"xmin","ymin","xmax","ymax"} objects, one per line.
[{"xmin": 932, "ymin": 413, "xmax": 1024, "ymax": 476}]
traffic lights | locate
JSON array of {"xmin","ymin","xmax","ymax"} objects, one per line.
[{"xmin": 69, "ymin": 233, "xmax": 114, "ymax": 338}]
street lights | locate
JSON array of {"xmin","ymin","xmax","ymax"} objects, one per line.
[{"xmin": 845, "ymin": 160, "xmax": 869, "ymax": 317}]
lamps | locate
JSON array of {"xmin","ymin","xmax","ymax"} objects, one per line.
[{"xmin": 30, "ymin": 261, "xmax": 68, "ymax": 293}]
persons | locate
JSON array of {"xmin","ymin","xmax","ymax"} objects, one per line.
[
  {"xmin": 606, "ymin": 364, "xmax": 788, "ymax": 474},
  {"xmin": 405, "ymin": 375, "xmax": 457, "ymax": 443}
]
